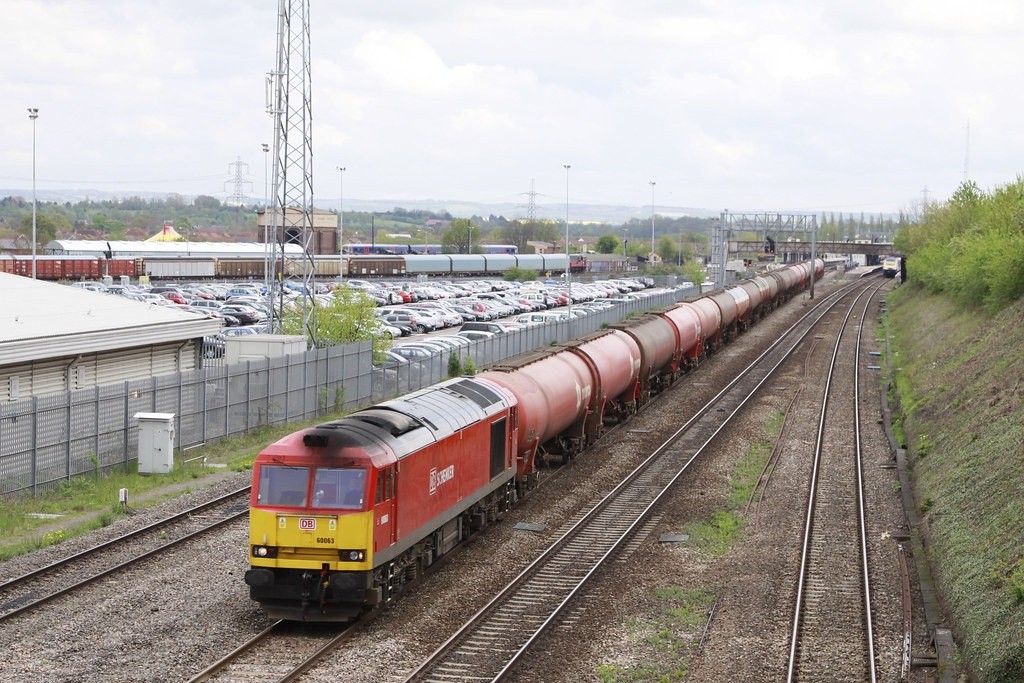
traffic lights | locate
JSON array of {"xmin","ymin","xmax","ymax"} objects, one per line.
[
  {"xmin": 748, "ymin": 260, "xmax": 752, "ymax": 268},
  {"xmin": 770, "ymin": 245, "xmax": 774, "ymax": 254}
]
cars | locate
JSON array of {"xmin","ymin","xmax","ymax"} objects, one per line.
[{"xmin": 72, "ymin": 277, "xmax": 694, "ymax": 393}]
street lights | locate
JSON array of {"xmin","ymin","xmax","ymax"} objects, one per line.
[
  {"xmin": 337, "ymin": 167, "xmax": 346, "ymax": 283},
  {"xmin": 564, "ymin": 165, "xmax": 571, "ymax": 284},
  {"xmin": 648, "ymin": 181, "xmax": 656, "ymax": 266},
  {"xmin": 262, "ymin": 143, "xmax": 269, "ymax": 286},
  {"xmin": 28, "ymin": 108, "xmax": 39, "ymax": 279},
  {"xmin": 827, "ymin": 232, "xmax": 835, "ymax": 240},
  {"xmin": 417, "ymin": 230, "xmax": 427, "ymax": 254},
  {"xmin": 467, "ymin": 227, "xmax": 475, "ymax": 254},
  {"xmin": 623, "ymin": 229, "xmax": 628, "ymax": 256},
  {"xmin": 679, "ymin": 229, "xmax": 682, "ymax": 266}
]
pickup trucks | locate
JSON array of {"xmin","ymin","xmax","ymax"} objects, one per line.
[{"xmin": 786, "ymin": 237, "xmax": 800, "ymax": 242}]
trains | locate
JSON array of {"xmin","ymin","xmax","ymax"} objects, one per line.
[
  {"xmin": 0, "ymin": 254, "xmax": 588, "ymax": 280},
  {"xmin": 883, "ymin": 257, "xmax": 901, "ymax": 278},
  {"xmin": 338, "ymin": 244, "xmax": 518, "ymax": 255},
  {"xmin": 244, "ymin": 258, "xmax": 824, "ymax": 623}
]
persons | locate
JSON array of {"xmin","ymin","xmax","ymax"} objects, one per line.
[{"xmin": 342, "ymin": 471, "xmax": 366, "ymax": 504}]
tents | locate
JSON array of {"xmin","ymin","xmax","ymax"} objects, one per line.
[{"xmin": 145, "ymin": 225, "xmax": 192, "ymax": 242}]
